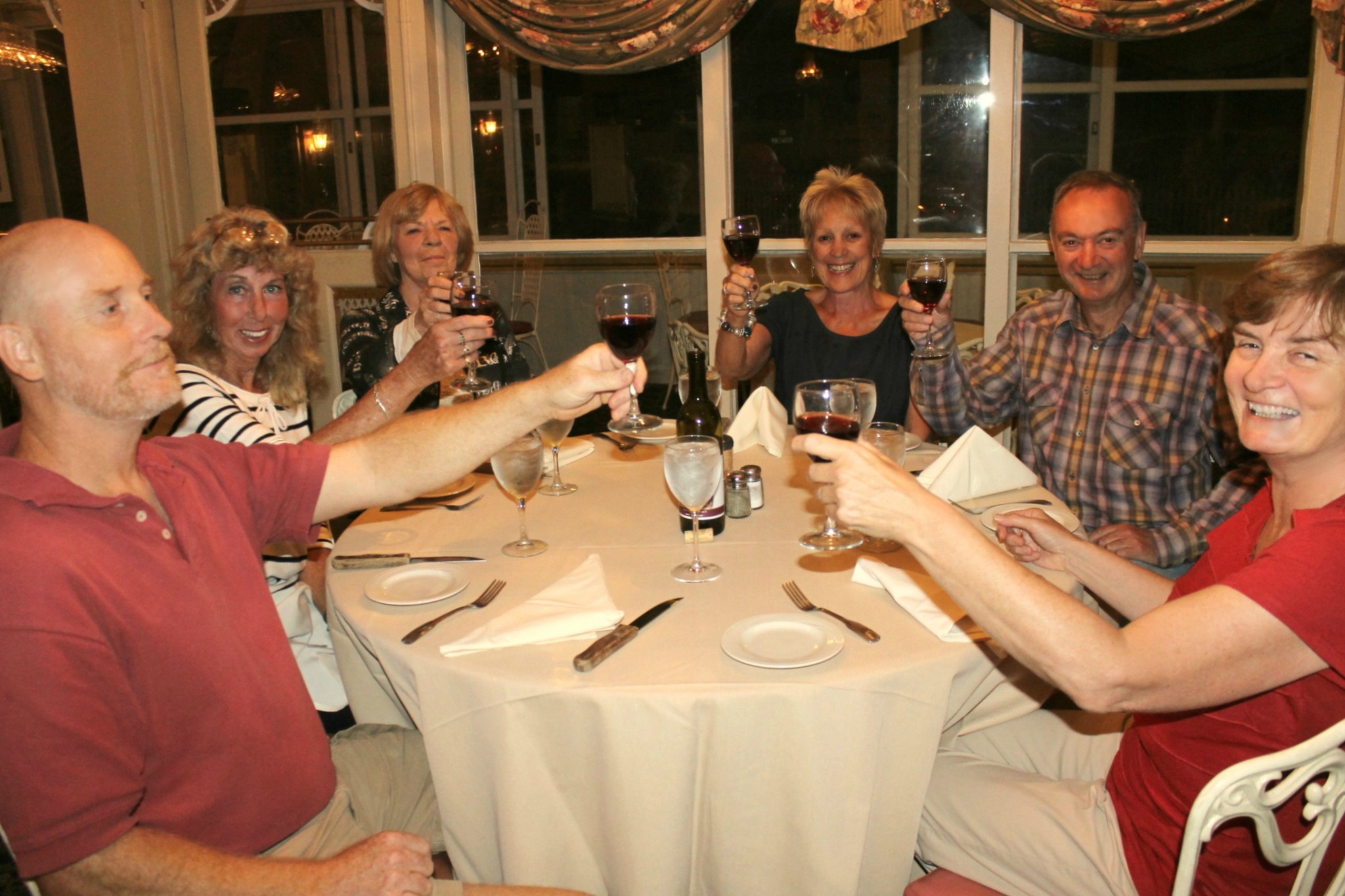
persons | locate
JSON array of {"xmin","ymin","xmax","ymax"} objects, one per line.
[
  {"xmin": 898, "ymin": 168, "xmax": 1272, "ymax": 583},
  {"xmin": 337, "ymin": 182, "xmax": 532, "ymax": 412},
  {"xmin": 788, "ymin": 242, "xmax": 1345, "ymax": 896},
  {"xmin": 714, "ymin": 168, "xmax": 934, "ymax": 444},
  {"xmin": 0, "ymin": 218, "xmax": 647, "ymax": 896},
  {"xmin": 139, "ymin": 207, "xmax": 496, "ymax": 734}
]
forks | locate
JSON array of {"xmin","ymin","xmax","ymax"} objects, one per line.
[
  {"xmin": 401, "ymin": 579, "xmax": 506, "ymax": 645},
  {"xmin": 781, "ymin": 580, "xmax": 881, "ymax": 643},
  {"xmin": 592, "ymin": 432, "xmax": 639, "ymax": 450},
  {"xmin": 379, "ymin": 495, "xmax": 482, "ymax": 511},
  {"xmin": 948, "ymin": 497, "xmax": 1052, "ymax": 514}
]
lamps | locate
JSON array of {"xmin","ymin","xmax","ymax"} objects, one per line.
[
  {"xmin": 484, "ymin": 110, "xmax": 497, "ymax": 134},
  {"xmin": 312, "ymin": 124, "xmax": 328, "ymax": 149}
]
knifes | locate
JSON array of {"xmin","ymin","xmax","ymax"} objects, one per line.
[
  {"xmin": 910, "ymin": 469, "xmax": 925, "ymax": 476},
  {"xmin": 572, "ymin": 597, "xmax": 685, "ymax": 673},
  {"xmin": 330, "ymin": 553, "xmax": 487, "ymax": 570}
]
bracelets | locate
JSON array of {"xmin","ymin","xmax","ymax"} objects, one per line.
[
  {"xmin": 718, "ymin": 306, "xmax": 757, "ymax": 337},
  {"xmin": 374, "ymin": 383, "xmax": 391, "ymax": 420}
]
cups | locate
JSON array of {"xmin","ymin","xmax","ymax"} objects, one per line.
[
  {"xmin": 677, "ymin": 364, "xmax": 723, "ymax": 410},
  {"xmin": 435, "ymin": 271, "xmax": 476, "ymax": 322},
  {"xmin": 830, "ymin": 378, "xmax": 877, "ymax": 436}
]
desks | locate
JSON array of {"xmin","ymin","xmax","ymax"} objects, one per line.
[{"xmin": 324, "ymin": 432, "xmax": 1102, "ymax": 896}]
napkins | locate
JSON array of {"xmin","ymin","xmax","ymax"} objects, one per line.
[
  {"xmin": 439, "ymin": 553, "xmax": 626, "ymax": 657},
  {"xmin": 725, "ymin": 386, "xmax": 789, "ymax": 458},
  {"xmin": 850, "ymin": 552, "xmax": 990, "ymax": 644},
  {"xmin": 544, "ymin": 434, "xmax": 594, "ymax": 471},
  {"xmin": 916, "ymin": 425, "xmax": 1040, "ymax": 506}
]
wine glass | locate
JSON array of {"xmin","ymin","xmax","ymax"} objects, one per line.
[
  {"xmin": 720, "ymin": 214, "xmax": 769, "ymax": 310},
  {"xmin": 790, "ymin": 378, "xmax": 865, "ymax": 552},
  {"xmin": 490, "ymin": 430, "xmax": 548, "ymax": 557},
  {"xmin": 451, "ymin": 275, "xmax": 499, "ymax": 392},
  {"xmin": 858, "ymin": 421, "xmax": 905, "ymax": 553},
  {"xmin": 535, "ymin": 418, "xmax": 579, "ymax": 497},
  {"xmin": 905, "ymin": 255, "xmax": 951, "ymax": 359},
  {"xmin": 594, "ymin": 282, "xmax": 664, "ymax": 433},
  {"xmin": 662, "ymin": 435, "xmax": 723, "ymax": 583}
]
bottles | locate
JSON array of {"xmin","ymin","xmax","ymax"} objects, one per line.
[
  {"xmin": 712, "ymin": 431, "xmax": 765, "ymax": 519},
  {"xmin": 674, "ymin": 349, "xmax": 724, "ymax": 536}
]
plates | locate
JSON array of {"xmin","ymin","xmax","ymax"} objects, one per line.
[
  {"xmin": 903, "ymin": 430, "xmax": 923, "ymax": 452},
  {"xmin": 980, "ymin": 503, "xmax": 1081, "ymax": 532},
  {"xmin": 607, "ymin": 418, "xmax": 678, "ymax": 444},
  {"xmin": 418, "ymin": 473, "xmax": 476, "ymax": 498},
  {"xmin": 363, "ymin": 561, "xmax": 470, "ymax": 606},
  {"xmin": 720, "ymin": 614, "xmax": 846, "ymax": 669}
]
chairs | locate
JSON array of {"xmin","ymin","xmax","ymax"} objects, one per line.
[
  {"xmin": 1172, "ymin": 717, "xmax": 1345, "ymax": 896},
  {"xmin": 506, "ymin": 198, "xmax": 549, "ymax": 373},
  {"xmin": 295, "ymin": 209, "xmax": 386, "ymax": 317},
  {"xmin": 956, "ymin": 287, "xmax": 1056, "ymax": 460},
  {"xmin": 653, "ymin": 256, "xmax": 825, "ymax": 421}
]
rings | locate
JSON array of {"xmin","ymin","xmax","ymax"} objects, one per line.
[
  {"xmin": 465, "ymin": 355, "xmax": 470, "ymax": 366},
  {"xmin": 457, "ymin": 330, "xmax": 466, "ymax": 346},
  {"xmin": 464, "ymin": 344, "xmax": 470, "ymax": 355}
]
